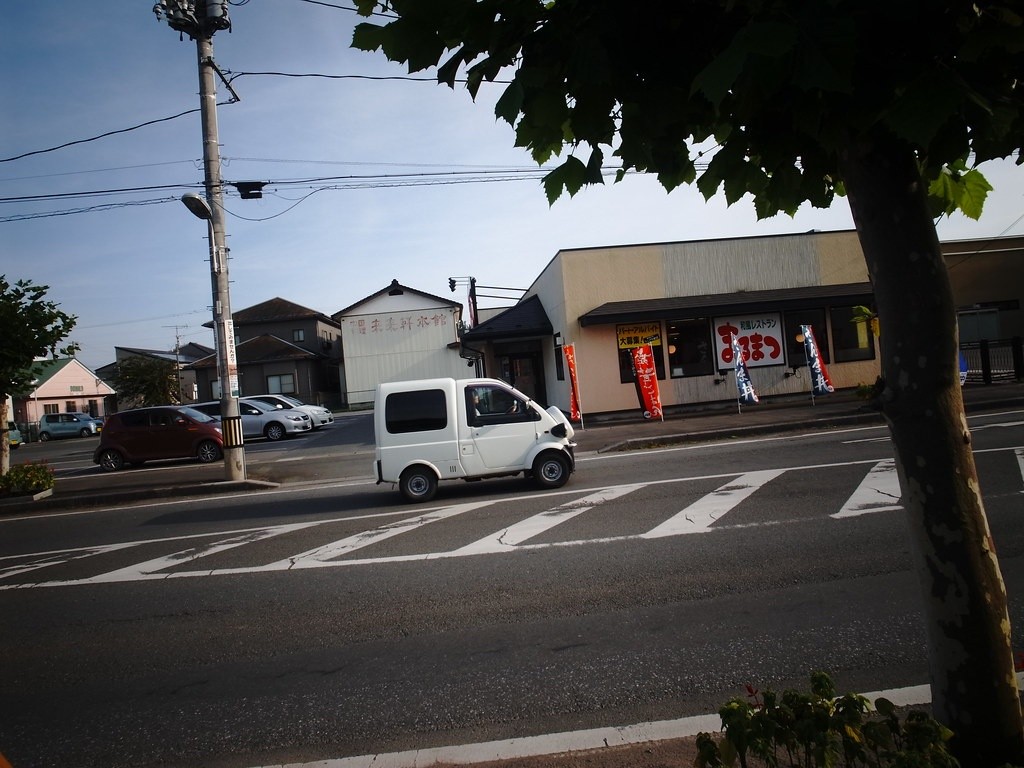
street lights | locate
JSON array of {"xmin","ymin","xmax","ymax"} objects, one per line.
[{"xmin": 181, "ymin": 191, "xmax": 247, "ymax": 484}]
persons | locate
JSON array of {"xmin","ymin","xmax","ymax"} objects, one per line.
[{"xmin": 515, "ymin": 367, "xmax": 536, "ymax": 413}]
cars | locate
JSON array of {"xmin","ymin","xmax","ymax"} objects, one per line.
[
  {"xmin": 183, "ymin": 394, "xmax": 335, "ymax": 442},
  {"xmin": 7, "ymin": 421, "xmax": 24, "ymax": 450},
  {"xmin": 93, "ymin": 404, "xmax": 226, "ymax": 473}
]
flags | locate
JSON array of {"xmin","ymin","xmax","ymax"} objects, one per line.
[
  {"xmin": 801, "ymin": 325, "xmax": 835, "ymax": 395},
  {"xmin": 628, "ymin": 344, "xmax": 664, "ymax": 419},
  {"xmin": 730, "ymin": 332, "xmax": 759, "ymax": 405},
  {"xmin": 563, "ymin": 344, "xmax": 580, "ymax": 420}
]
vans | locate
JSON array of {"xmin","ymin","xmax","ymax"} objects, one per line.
[
  {"xmin": 373, "ymin": 376, "xmax": 578, "ymax": 503},
  {"xmin": 36, "ymin": 411, "xmax": 104, "ymax": 442}
]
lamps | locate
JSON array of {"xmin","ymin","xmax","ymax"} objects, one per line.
[
  {"xmin": 714, "ymin": 369, "xmax": 727, "ymax": 385},
  {"xmin": 784, "ymin": 364, "xmax": 799, "ymax": 378}
]
